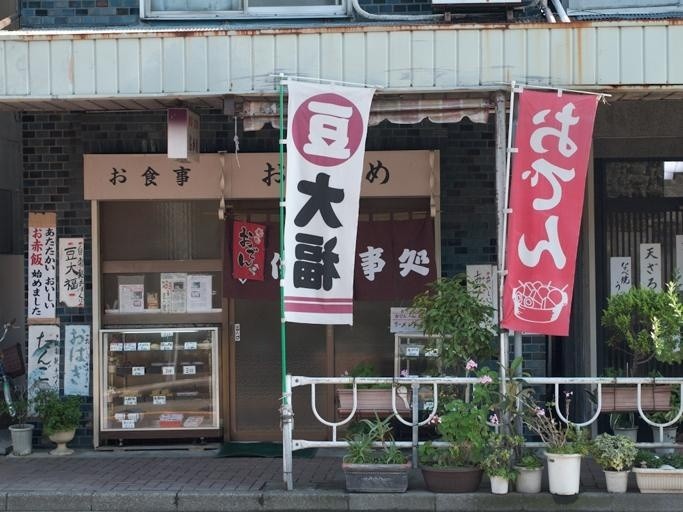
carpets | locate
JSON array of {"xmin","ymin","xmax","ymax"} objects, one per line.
[{"xmin": 212, "ymin": 441, "xmax": 317, "ymax": 460}]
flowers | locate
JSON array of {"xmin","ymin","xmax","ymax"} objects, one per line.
[{"xmin": 462, "ymin": 358, "xmax": 510, "ymax": 427}]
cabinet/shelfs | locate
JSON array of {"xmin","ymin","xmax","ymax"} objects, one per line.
[{"xmin": 98, "ymin": 329, "xmax": 219, "ymax": 429}]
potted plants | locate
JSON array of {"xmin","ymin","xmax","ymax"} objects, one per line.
[
  {"xmin": 478, "ymin": 410, "xmax": 683, "ymax": 495},
  {"xmin": 1, "ymin": 389, "xmax": 84, "ymax": 456},
  {"xmin": 339, "ymin": 399, "xmax": 489, "ymax": 493},
  {"xmin": 336, "ymin": 358, "xmax": 412, "ymax": 413}
]
